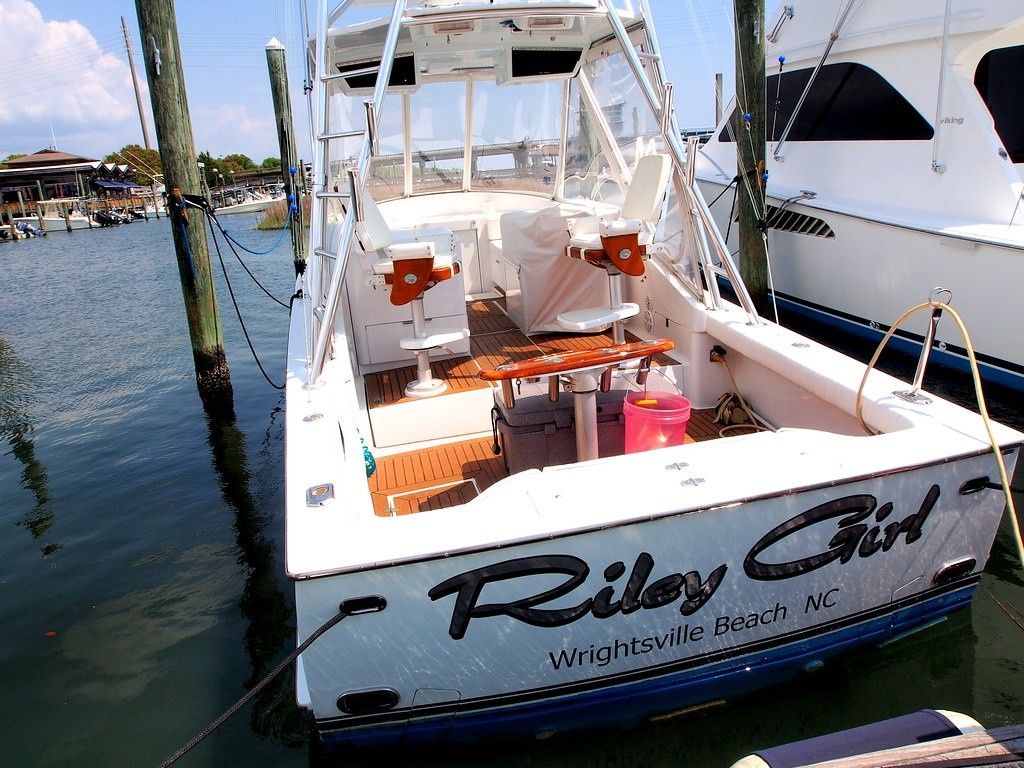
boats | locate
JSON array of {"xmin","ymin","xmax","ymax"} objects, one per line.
[
  {"xmin": 0, "ymin": 225, "xmax": 35, "ymax": 240},
  {"xmin": 12, "ymin": 199, "xmax": 101, "ymax": 232},
  {"xmin": 280, "ymin": 0, "xmax": 1024, "ymax": 752},
  {"xmin": 145, "ymin": 201, "xmax": 170, "ymax": 217},
  {"xmin": 91, "ymin": 206, "xmax": 146, "ymax": 226},
  {"xmin": 214, "ymin": 191, "xmax": 282, "ymax": 215},
  {"xmin": 694, "ymin": 0, "xmax": 1024, "ymax": 389}
]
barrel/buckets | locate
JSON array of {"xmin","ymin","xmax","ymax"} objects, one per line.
[{"xmin": 623, "ymin": 367, "xmax": 691, "ymax": 455}]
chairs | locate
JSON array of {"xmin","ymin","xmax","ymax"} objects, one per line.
[
  {"xmin": 477, "ymin": 338, "xmax": 685, "ymax": 476},
  {"xmin": 557, "ymin": 155, "xmax": 673, "ymax": 368},
  {"xmin": 333, "ymin": 182, "xmax": 470, "ymax": 398}
]
